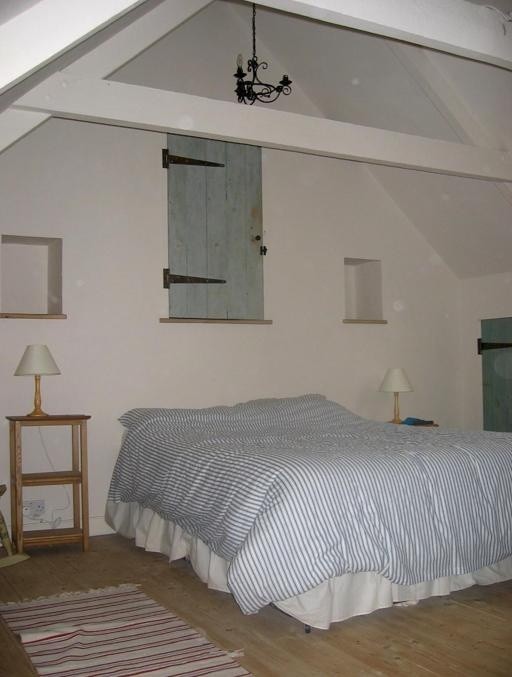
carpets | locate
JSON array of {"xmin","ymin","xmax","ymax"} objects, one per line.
[{"xmin": 0, "ymin": 581, "xmax": 261, "ymax": 675}]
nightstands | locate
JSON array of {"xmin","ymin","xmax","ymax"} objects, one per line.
[
  {"xmin": 7, "ymin": 413, "xmax": 95, "ymax": 553},
  {"xmin": 408, "ymin": 421, "xmax": 440, "ymax": 427}
]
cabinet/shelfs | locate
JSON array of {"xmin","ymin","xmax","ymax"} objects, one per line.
[{"xmin": 475, "ymin": 315, "xmax": 511, "ymax": 435}]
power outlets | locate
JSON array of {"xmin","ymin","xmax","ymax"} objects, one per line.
[{"xmin": 21, "ymin": 501, "xmax": 46, "ymax": 516}]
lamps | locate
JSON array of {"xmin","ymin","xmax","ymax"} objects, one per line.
[
  {"xmin": 12, "ymin": 341, "xmax": 65, "ymax": 418},
  {"xmin": 376, "ymin": 365, "xmax": 415, "ymax": 424},
  {"xmin": 231, "ymin": 1, "xmax": 295, "ymax": 104}
]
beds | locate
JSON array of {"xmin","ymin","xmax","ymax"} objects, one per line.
[{"xmin": 103, "ymin": 393, "xmax": 511, "ymax": 632}]
